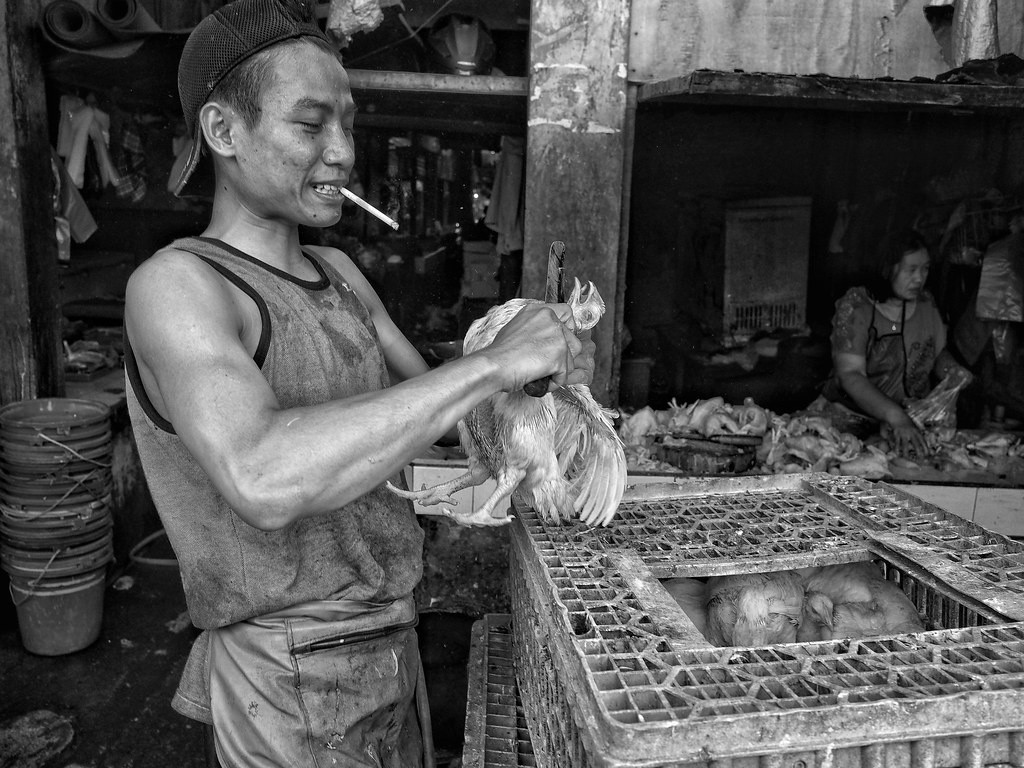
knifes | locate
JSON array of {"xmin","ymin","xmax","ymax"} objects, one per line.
[{"xmin": 523, "ymin": 240, "xmax": 566, "ymax": 398}]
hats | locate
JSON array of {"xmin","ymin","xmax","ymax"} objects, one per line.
[{"xmin": 175, "ymin": 0, "xmax": 333, "ymax": 200}]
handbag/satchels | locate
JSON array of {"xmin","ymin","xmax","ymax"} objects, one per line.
[{"xmin": 904, "ymin": 367, "xmax": 967, "ymax": 445}]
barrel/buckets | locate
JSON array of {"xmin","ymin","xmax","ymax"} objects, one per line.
[{"xmin": 1, "ymin": 398, "xmax": 111, "ymax": 655}]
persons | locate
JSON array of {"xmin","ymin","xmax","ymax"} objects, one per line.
[
  {"xmin": 806, "ymin": 229, "xmax": 975, "ymax": 464},
  {"xmin": 121, "ymin": 0, "xmax": 584, "ymax": 767}
]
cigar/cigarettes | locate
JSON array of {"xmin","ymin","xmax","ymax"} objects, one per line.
[{"xmin": 337, "ymin": 185, "xmax": 400, "ymax": 232}]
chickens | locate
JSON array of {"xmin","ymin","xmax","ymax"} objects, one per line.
[
  {"xmin": 658, "ymin": 560, "xmax": 926, "ymax": 647},
  {"xmin": 612, "ymin": 396, "xmax": 892, "ymax": 481},
  {"xmin": 384, "ymin": 273, "xmax": 629, "ymax": 529}
]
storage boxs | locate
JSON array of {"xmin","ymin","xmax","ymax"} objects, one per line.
[
  {"xmin": 460, "ymin": 609, "xmax": 538, "ymax": 768},
  {"xmin": 511, "ymin": 465, "xmax": 1024, "ymax": 767},
  {"xmin": 1, "ymin": 395, "xmax": 119, "ymax": 659}
]
